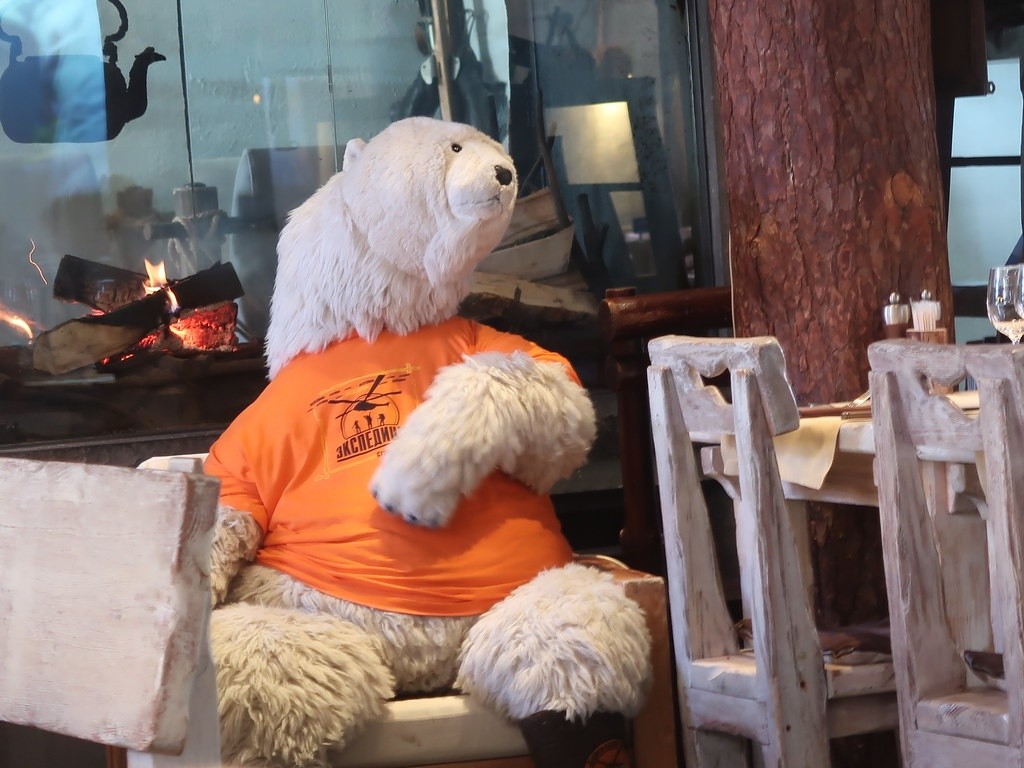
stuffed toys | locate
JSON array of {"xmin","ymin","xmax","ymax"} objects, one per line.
[{"xmin": 204, "ymin": 115, "xmax": 652, "ymax": 768}]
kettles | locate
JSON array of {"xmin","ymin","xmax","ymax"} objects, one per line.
[{"xmin": 0, "ymin": 0, "xmax": 166, "ymax": 144}]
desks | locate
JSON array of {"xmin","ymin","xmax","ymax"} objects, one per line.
[{"xmin": 700, "ymin": 391, "xmax": 982, "ymax": 653}]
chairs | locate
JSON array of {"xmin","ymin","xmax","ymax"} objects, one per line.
[
  {"xmin": 0, "ymin": 454, "xmax": 677, "ymax": 768},
  {"xmin": 647, "ymin": 336, "xmax": 1024, "ymax": 768}
]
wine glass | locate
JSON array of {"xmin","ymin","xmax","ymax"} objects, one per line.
[{"xmin": 986, "ymin": 265, "xmax": 1024, "ymax": 344}]
